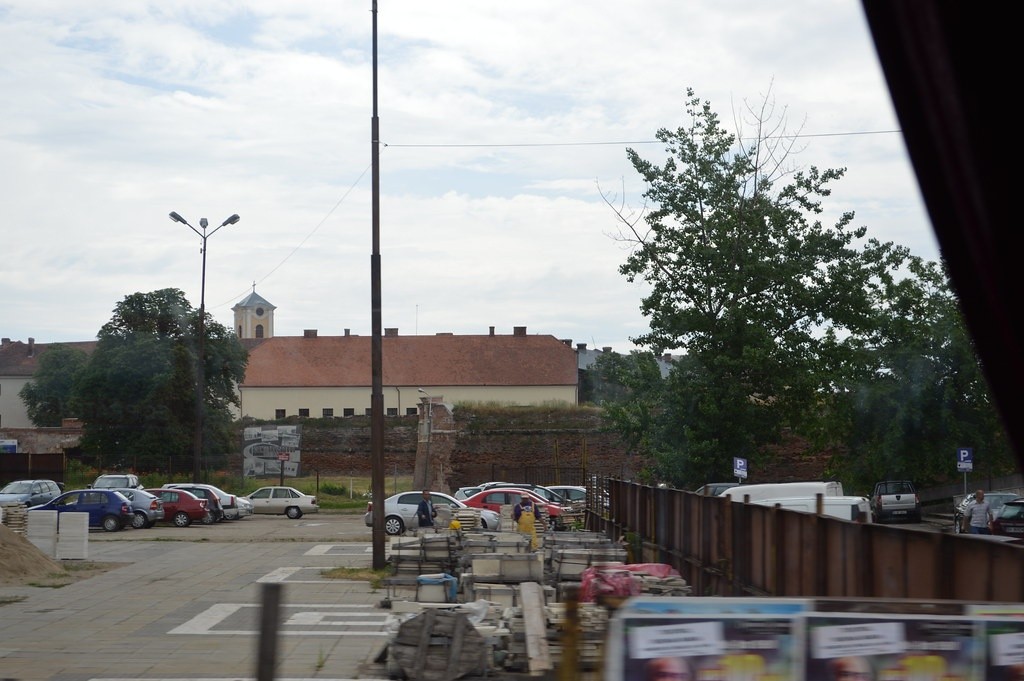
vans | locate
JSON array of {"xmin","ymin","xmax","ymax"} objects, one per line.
[{"xmin": 695, "ymin": 481, "xmax": 874, "ymax": 525}]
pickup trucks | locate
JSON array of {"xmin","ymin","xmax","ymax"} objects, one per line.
[{"xmin": 867, "ymin": 481, "xmax": 922, "ymax": 523}]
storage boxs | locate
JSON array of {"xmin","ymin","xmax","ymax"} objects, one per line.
[{"xmin": 386, "ymin": 504, "xmax": 694, "ymax": 681}]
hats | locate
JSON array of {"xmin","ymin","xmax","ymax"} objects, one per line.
[{"xmin": 521, "ymin": 492, "xmax": 529, "ymax": 498}]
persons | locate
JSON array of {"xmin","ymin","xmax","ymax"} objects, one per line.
[
  {"xmin": 962, "ymin": 490, "xmax": 994, "ymax": 536},
  {"xmin": 417, "ymin": 489, "xmax": 438, "ymax": 527},
  {"xmin": 514, "ymin": 492, "xmax": 548, "ymax": 552}
]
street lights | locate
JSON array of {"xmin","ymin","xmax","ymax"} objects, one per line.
[
  {"xmin": 417, "ymin": 387, "xmax": 430, "ymax": 492},
  {"xmin": 170, "ymin": 210, "xmax": 241, "ymax": 482}
]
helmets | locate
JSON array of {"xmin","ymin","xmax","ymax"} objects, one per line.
[{"xmin": 449, "ymin": 521, "xmax": 461, "ymax": 530}]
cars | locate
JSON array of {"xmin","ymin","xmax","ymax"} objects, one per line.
[
  {"xmin": 531, "ymin": 484, "xmax": 610, "ymax": 510},
  {"xmin": 224, "ymin": 497, "xmax": 254, "ymax": 521},
  {"xmin": 479, "ymin": 482, "xmax": 576, "ymax": 504},
  {"xmin": 168, "ymin": 486, "xmax": 225, "ymax": 526},
  {"xmin": 453, "ymin": 487, "xmax": 485, "ymax": 501},
  {"xmin": 143, "ymin": 489, "xmax": 210, "ymax": 526},
  {"xmin": 461, "ymin": 489, "xmax": 572, "ymax": 524},
  {"xmin": 0, "ymin": 478, "xmax": 62, "ymax": 511},
  {"xmin": 363, "ymin": 490, "xmax": 504, "ymax": 536},
  {"xmin": 113, "ymin": 488, "xmax": 165, "ymax": 529},
  {"xmin": 952, "ymin": 491, "xmax": 1019, "ymax": 534},
  {"xmin": 994, "ymin": 496, "xmax": 1024, "ymax": 538},
  {"xmin": 87, "ymin": 473, "xmax": 139, "ymax": 490},
  {"xmin": 160, "ymin": 483, "xmax": 238, "ymax": 523},
  {"xmin": 27, "ymin": 489, "xmax": 135, "ymax": 532},
  {"xmin": 239, "ymin": 486, "xmax": 319, "ymax": 520}
]
